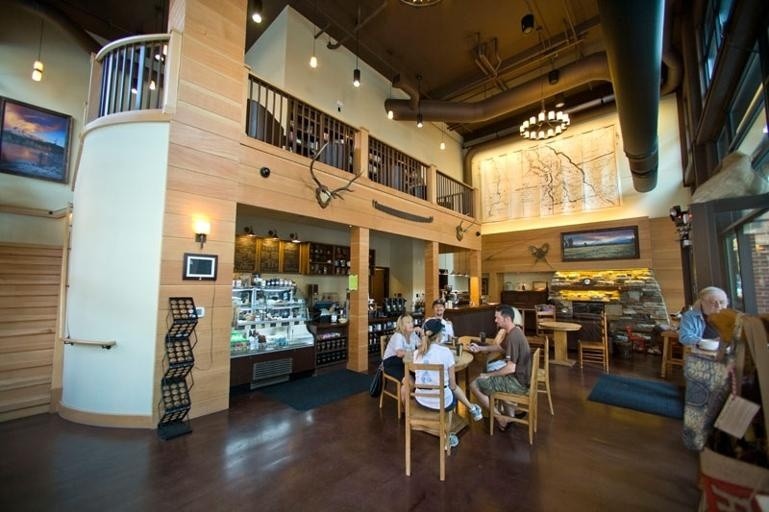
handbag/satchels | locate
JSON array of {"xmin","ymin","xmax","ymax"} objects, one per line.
[{"xmin": 369, "ymin": 367, "xmax": 388, "ymax": 397}]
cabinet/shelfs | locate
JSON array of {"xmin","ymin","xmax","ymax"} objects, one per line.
[
  {"xmin": 231, "ymin": 283, "xmax": 317, "ymax": 391},
  {"xmin": 549, "ymin": 270, "xmax": 674, "ymax": 353},
  {"xmin": 299, "ymin": 240, "xmax": 335, "ymax": 276},
  {"xmin": 335, "ymin": 245, "xmax": 376, "ymax": 277},
  {"xmin": 306, "ymin": 311, "xmax": 423, "ymax": 369}
]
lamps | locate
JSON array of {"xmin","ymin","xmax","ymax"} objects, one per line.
[
  {"xmin": 191, "ymin": 216, "xmax": 211, "ymax": 249},
  {"xmin": 547, "ymin": 68, "xmax": 561, "ymax": 87},
  {"xmin": 30, "ymin": 10, "xmax": 47, "ymax": 84},
  {"xmin": 520, "ymin": 13, "xmax": 538, "ymax": 37},
  {"xmin": 438, "ymin": 243, "xmax": 472, "ymax": 278},
  {"xmin": 131, "ymin": 46, "xmax": 160, "ymax": 94},
  {"xmin": 519, "ymin": 26, "xmax": 571, "ymax": 141},
  {"xmin": 251, "ymin": 0, "xmax": 447, "ymax": 151}
]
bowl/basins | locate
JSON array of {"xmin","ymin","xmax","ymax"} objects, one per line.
[{"xmin": 699, "ymin": 338, "xmax": 719, "ymax": 351}]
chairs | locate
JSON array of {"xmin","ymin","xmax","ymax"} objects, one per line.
[
  {"xmin": 246, "ymin": 99, "xmax": 284, "ymax": 148},
  {"xmin": 317, "ymin": 143, "xmax": 351, "ymax": 171},
  {"xmin": 374, "ymin": 167, "xmax": 407, "ymax": 193},
  {"xmin": 408, "ymin": 186, "xmax": 426, "ymax": 200}
]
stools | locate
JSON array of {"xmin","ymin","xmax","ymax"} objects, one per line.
[{"xmin": 661, "ymin": 331, "xmax": 687, "ymax": 380}]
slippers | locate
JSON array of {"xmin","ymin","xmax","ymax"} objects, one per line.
[
  {"xmin": 497, "ymin": 422, "xmax": 512, "ymax": 431},
  {"xmin": 511, "ymin": 410, "xmax": 526, "ymax": 418}
]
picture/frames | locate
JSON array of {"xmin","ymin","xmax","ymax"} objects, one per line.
[
  {"xmin": 560, "ymin": 225, "xmax": 639, "ymax": 262},
  {"xmin": 0, "ymin": 96, "xmax": 73, "ymax": 185}
]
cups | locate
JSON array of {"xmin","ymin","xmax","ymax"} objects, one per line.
[
  {"xmin": 452, "ymin": 336, "xmax": 457, "ymax": 348},
  {"xmin": 455, "ymin": 344, "xmax": 463, "ymax": 357},
  {"xmin": 480, "ymin": 332, "xmax": 486, "ymax": 345}
]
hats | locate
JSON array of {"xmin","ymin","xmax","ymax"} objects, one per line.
[
  {"xmin": 421, "ymin": 318, "xmax": 447, "ymax": 337},
  {"xmin": 432, "ymin": 299, "xmax": 447, "ymax": 307}
]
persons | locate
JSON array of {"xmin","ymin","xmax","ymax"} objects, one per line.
[
  {"xmin": 678, "ymin": 287, "xmax": 737, "ymax": 450},
  {"xmin": 413, "ymin": 319, "xmax": 483, "ymax": 450},
  {"xmin": 384, "ymin": 314, "xmax": 422, "ymax": 407},
  {"xmin": 424, "ymin": 300, "xmax": 454, "ymax": 345},
  {"xmin": 464, "ymin": 304, "xmax": 531, "ymax": 432}
]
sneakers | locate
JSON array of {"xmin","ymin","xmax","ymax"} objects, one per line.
[
  {"xmin": 445, "ymin": 431, "xmax": 460, "ymax": 450},
  {"xmin": 470, "ymin": 402, "xmax": 482, "ymax": 421}
]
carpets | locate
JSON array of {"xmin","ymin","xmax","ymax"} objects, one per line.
[
  {"xmin": 587, "ymin": 374, "xmax": 685, "ymax": 421},
  {"xmin": 262, "ymin": 368, "xmax": 373, "ymax": 412}
]
